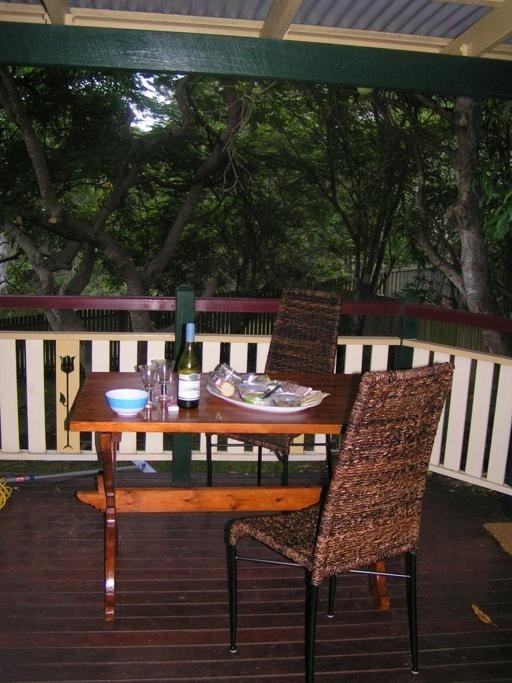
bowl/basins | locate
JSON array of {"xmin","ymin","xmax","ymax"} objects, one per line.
[{"xmin": 105, "ymin": 388, "xmax": 150, "ymax": 416}]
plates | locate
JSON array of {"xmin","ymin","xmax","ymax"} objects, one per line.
[{"xmin": 207, "ymin": 380, "xmax": 321, "ymax": 413}]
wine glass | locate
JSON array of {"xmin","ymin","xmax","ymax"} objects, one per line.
[{"xmin": 134, "ymin": 359, "xmax": 176, "ymax": 412}]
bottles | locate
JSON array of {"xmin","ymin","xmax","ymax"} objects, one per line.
[{"xmin": 177, "ymin": 323, "xmax": 200, "ymax": 409}]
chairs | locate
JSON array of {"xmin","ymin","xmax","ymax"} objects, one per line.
[
  {"xmin": 206, "ymin": 290, "xmax": 342, "ymax": 492},
  {"xmin": 227, "ymin": 361, "xmax": 455, "ymax": 681}
]
